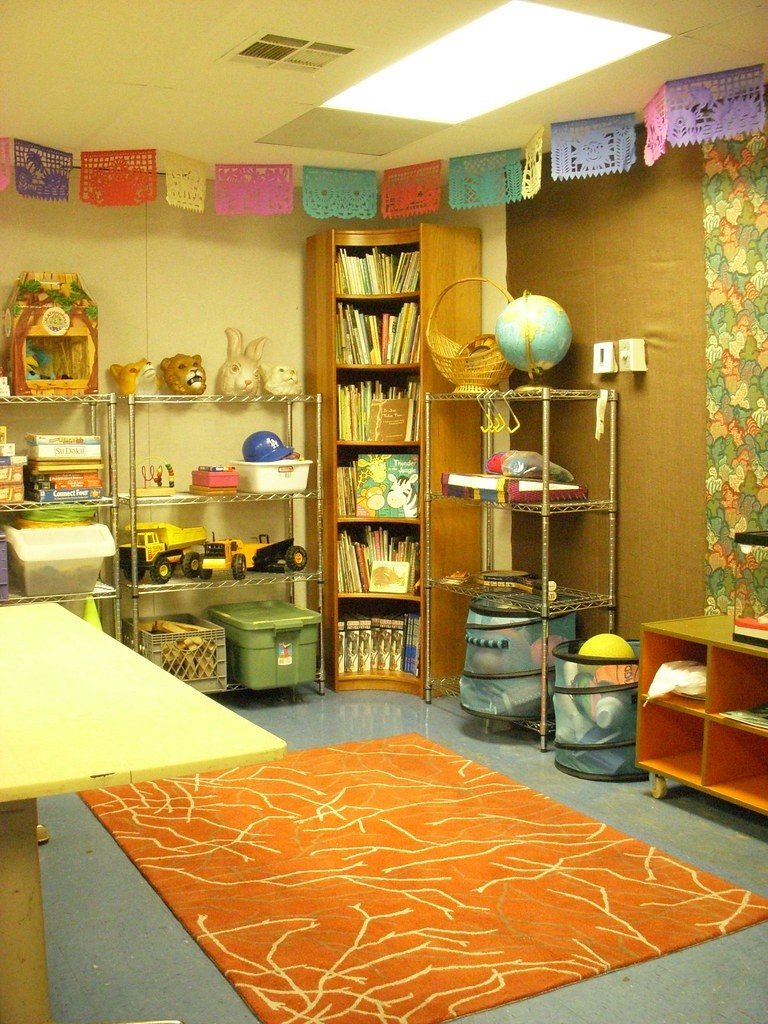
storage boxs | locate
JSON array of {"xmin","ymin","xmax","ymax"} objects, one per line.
[
  {"xmin": 211, "ymin": 599, "xmax": 322, "ymax": 691},
  {"xmin": 229, "ymin": 460, "xmax": 312, "ymax": 495},
  {"xmin": 191, "ymin": 470, "xmax": 238, "ymax": 489},
  {"xmin": 439, "ymin": 473, "xmax": 590, "ymax": 502},
  {"xmin": 4, "ymin": 521, "xmax": 119, "ymax": 598},
  {"xmin": 122, "ymin": 613, "xmax": 228, "ymax": 695}
]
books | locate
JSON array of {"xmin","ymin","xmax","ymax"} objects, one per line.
[
  {"xmin": 338, "ymin": 526, "xmax": 421, "ymax": 596},
  {"xmin": 336, "ymin": 612, "xmax": 419, "ymax": 678},
  {"xmin": 337, "ymin": 376, "xmax": 422, "ymax": 442},
  {"xmin": 335, "ymin": 302, "xmax": 420, "ymax": 365},
  {"xmin": 336, "ymin": 454, "xmax": 420, "ymax": 518},
  {"xmin": 335, "ymin": 246, "xmax": 420, "ymax": 295}
]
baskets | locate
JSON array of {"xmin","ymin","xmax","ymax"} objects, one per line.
[
  {"xmin": 425, "ymin": 277, "xmax": 523, "ymax": 394},
  {"xmin": 121, "ymin": 611, "xmax": 228, "ymax": 694}
]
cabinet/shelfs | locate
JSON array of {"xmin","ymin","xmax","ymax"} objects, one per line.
[
  {"xmin": 0, "ymin": 391, "xmax": 124, "ymax": 641},
  {"xmin": 425, "ymin": 390, "xmax": 617, "ymax": 754},
  {"xmin": 631, "ymin": 613, "xmax": 768, "ymax": 816},
  {"xmin": 304, "ymin": 224, "xmax": 488, "ymax": 701},
  {"xmin": 120, "ymin": 392, "xmax": 328, "ymax": 703}
]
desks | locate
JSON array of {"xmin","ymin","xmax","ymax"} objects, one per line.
[{"xmin": 0, "ymin": 602, "xmax": 287, "ymax": 1024}]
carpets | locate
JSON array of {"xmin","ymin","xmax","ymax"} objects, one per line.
[{"xmin": 75, "ymin": 734, "xmax": 768, "ymax": 1024}]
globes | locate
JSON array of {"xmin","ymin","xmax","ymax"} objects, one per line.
[{"xmin": 495, "ymin": 286, "xmax": 574, "ymax": 392}]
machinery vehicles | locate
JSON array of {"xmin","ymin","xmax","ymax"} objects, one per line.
[
  {"xmin": 196, "ymin": 529, "xmax": 309, "ymax": 581},
  {"xmin": 119, "ymin": 519, "xmax": 207, "ymax": 585}
]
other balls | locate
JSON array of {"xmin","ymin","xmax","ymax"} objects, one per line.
[{"xmin": 575, "ymin": 633, "xmax": 634, "ymax": 675}]
